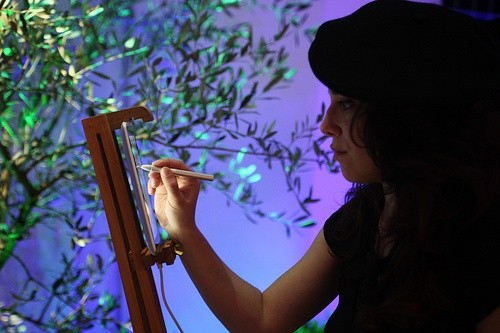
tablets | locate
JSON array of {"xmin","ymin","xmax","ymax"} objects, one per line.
[{"xmin": 121, "ymin": 122, "xmax": 162, "ymax": 256}]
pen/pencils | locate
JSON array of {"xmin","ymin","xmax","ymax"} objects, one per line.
[{"xmin": 136, "ymin": 162, "xmax": 214, "ymax": 182}]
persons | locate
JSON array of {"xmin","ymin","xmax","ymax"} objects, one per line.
[{"xmin": 147, "ymin": 0, "xmax": 500, "ymax": 333}]
